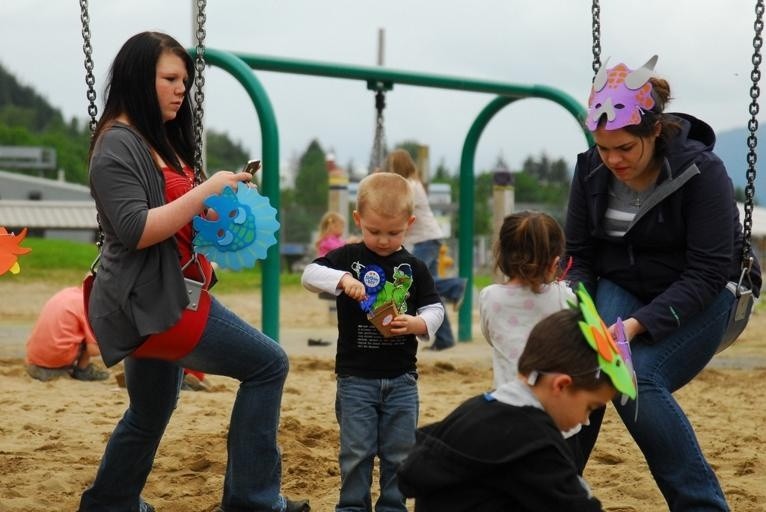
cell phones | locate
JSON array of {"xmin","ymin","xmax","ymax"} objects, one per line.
[{"xmin": 239, "ymin": 160, "xmax": 261, "ymax": 184}]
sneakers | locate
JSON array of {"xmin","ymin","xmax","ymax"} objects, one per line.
[
  {"xmin": 454, "ymin": 278, "xmax": 468, "ymax": 312},
  {"xmin": 72, "ymin": 363, "xmax": 108, "ymax": 380},
  {"xmin": 423, "ymin": 343, "xmax": 446, "ymax": 350},
  {"xmin": 286, "ymin": 500, "xmax": 311, "ymax": 512}
]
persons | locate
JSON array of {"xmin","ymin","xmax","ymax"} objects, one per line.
[
  {"xmin": 315, "ymin": 211, "xmax": 358, "ymax": 257},
  {"xmin": 397, "ymin": 308, "xmax": 618, "ymax": 512},
  {"xmin": 25, "ymin": 269, "xmax": 109, "ymax": 383},
  {"xmin": 561, "ymin": 76, "xmax": 762, "ymax": 512},
  {"xmin": 301, "ymin": 172, "xmax": 445, "ymax": 512},
  {"xmin": 479, "ymin": 210, "xmax": 591, "ymax": 477},
  {"xmin": 75, "ymin": 32, "xmax": 311, "ymax": 512},
  {"xmin": 384, "ymin": 149, "xmax": 468, "ymax": 350}
]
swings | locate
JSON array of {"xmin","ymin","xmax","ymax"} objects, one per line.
[
  {"xmin": 81, "ymin": 0, "xmax": 214, "ymax": 361},
  {"xmin": 592, "ymin": 0, "xmax": 765, "ymax": 354}
]
